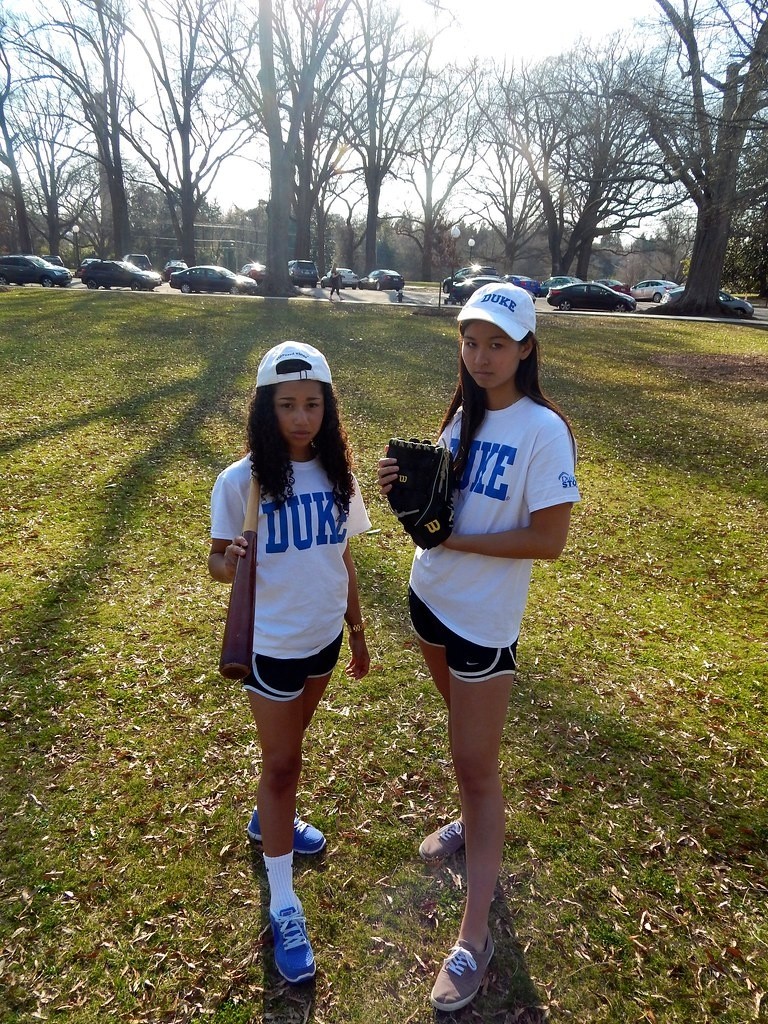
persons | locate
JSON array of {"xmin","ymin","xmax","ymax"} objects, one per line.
[
  {"xmin": 377, "ymin": 282, "xmax": 580, "ymax": 1010},
  {"xmin": 209, "ymin": 340, "xmax": 372, "ymax": 983},
  {"xmin": 329, "ymin": 263, "xmax": 345, "ymax": 300}
]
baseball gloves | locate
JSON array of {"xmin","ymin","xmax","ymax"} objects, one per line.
[{"xmin": 383, "ymin": 434, "xmax": 461, "ymax": 551}]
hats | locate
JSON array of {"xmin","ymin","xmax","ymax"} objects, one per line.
[
  {"xmin": 255, "ymin": 339, "xmax": 332, "ymax": 388},
  {"xmin": 456, "ymin": 282, "xmax": 537, "ymax": 341}
]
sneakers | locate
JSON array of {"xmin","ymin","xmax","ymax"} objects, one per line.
[
  {"xmin": 247, "ymin": 804, "xmax": 327, "ymax": 854},
  {"xmin": 419, "ymin": 817, "xmax": 465, "ymax": 861},
  {"xmin": 430, "ymin": 926, "xmax": 495, "ymax": 1011},
  {"xmin": 269, "ymin": 894, "xmax": 316, "ymax": 982}
]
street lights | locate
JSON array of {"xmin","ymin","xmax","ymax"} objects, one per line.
[
  {"xmin": 468, "ymin": 238, "xmax": 476, "ymax": 267},
  {"xmin": 451, "ymin": 225, "xmax": 460, "ymax": 286},
  {"xmin": 73, "ymin": 225, "xmax": 80, "ymax": 266}
]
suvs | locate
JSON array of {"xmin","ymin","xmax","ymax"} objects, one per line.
[
  {"xmin": 124, "ymin": 254, "xmax": 153, "ymax": 272},
  {"xmin": 442, "ymin": 266, "xmax": 501, "ymax": 294},
  {"xmin": 288, "ymin": 260, "xmax": 319, "ymax": 288}
]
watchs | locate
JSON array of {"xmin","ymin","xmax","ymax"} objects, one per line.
[{"xmin": 346, "ymin": 624, "xmax": 364, "ymax": 634}]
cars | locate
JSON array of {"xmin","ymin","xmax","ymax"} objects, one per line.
[
  {"xmin": 169, "ymin": 265, "xmax": 258, "ymax": 295},
  {"xmin": 241, "ymin": 263, "xmax": 266, "ymax": 282},
  {"xmin": 546, "ymin": 279, "xmax": 754, "ymax": 318},
  {"xmin": 81, "ymin": 260, "xmax": 162, "ymax": 291},
  {"xmin": 501, "ymin": 274, "xmax": 541, "ymax": 295},
  {"xmin": 321, "ymin": 268, "xmax": 359, "ymax": 290},
  {"xmin": 162, "ymin": 260, "xmax": 188, "ymax": 282},
  {"xmin": 451, "ymin": 278, "xmax": 537, "ymax": 306},
  {"xmin": 41, "ymin": 254, "xmax": 64, "ymax": 267},
  {"xmin": 79, "ymin": 259, "xmax": 101, "ymax": 275},
  {"xmin": 1, "ymin": 255, "xmax": 72, "ymax": 287},
  {"xmin": 540, "ymin": 276, "xmax": 586, "ymax": 297},
  {"xmin": 358, "ymin": 269, "xmax": 405, "ymax": 291}
]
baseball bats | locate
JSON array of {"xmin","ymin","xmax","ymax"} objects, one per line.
[{"xmin": 217, "ymin": 476, "xmax": 260, "ymax": 681}]
